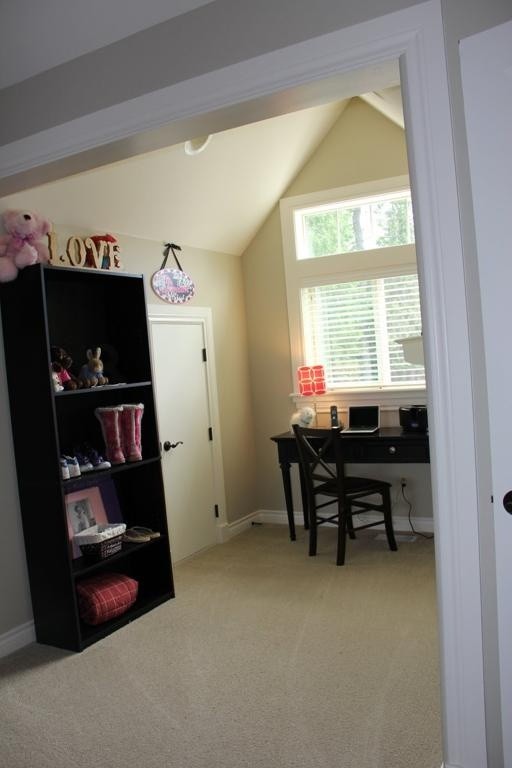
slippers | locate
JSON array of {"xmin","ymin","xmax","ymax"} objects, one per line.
[
  {"xmin": 130, "ymin": 526, "xmax": 160, "ymax": 539},
  {"xmin": 122, "ymin": 529, "xmax": 150, "ymax": 543}
]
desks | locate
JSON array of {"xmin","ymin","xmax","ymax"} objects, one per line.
[{"xmin": 268, "ymin": 428, "xmax": 431, "ymax": 541}]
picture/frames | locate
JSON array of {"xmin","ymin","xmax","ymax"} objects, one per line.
[{"xmin": 63, "ymin": 487, "xmax": 112, "ymax": 558}]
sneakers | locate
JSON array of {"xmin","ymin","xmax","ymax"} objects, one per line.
[
  {"xmin": 74, "ymin": 451, "xmax": 93, "ymax": 473},
  {"xmin": 62, "ymin": 455, "xmax": 81, "ymax": 477},
  {"xmin": 60, "ymin": 459, "xmax": 70, "ymax": 480},
  {"xmin": 84, "ymin": 448, "xmax": 111, "ymax": 470}
]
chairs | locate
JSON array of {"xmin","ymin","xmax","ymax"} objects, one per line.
[{"xmin": 291, "ymin": 424, "xmax": 398, "ymax": 566}]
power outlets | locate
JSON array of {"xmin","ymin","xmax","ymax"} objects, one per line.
[{"xmin": 395, "ymin": 476, "xmax": 415, "ymax": 500}]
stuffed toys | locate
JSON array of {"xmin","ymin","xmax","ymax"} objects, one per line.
[
  {"xmin": 51, "ymin": 345, "xmax": 83, "ymax": 391},
  {"xmin": 1, "ymin": 209, "xmax": 53, "ymax": 282},
  {"xmin": 52, "ymin": 372, "xmax": 64, "ymax": 393},
  {"xmin": 289, "ymin": 406, "xmax": 317, "ymax": 435},
  {"xmin": 78, "ymin": 346, "xmax": 110, "ymax": 387}
]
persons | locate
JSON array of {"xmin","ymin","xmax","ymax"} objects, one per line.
[{"xmin": 74, "ymin": 506, "xmax": 90, "ymax": 531}]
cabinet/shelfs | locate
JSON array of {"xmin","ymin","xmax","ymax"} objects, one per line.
[{"xmin": 1, "ymin": 262, "xmax": 176, "ymax": 652}]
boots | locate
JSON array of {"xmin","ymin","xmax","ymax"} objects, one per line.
[
  {"xmin": 95, "ymin": 407, "xmax": 125, "ymax": 464},
  {"xmin": 120, "ymin": 402, "xmax": 144, "ymax": 462}
]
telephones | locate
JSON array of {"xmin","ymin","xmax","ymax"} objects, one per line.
[{"xmin": 331, "ymin": 406, "xmax": 342, "ymax": 433}]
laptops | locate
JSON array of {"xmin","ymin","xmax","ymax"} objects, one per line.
[{"xmin": 339, "ymin": 405, "xmax": 382, "ymax": 434}]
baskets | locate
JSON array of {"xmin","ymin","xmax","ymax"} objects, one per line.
[{"xmin": 79, "ymin": 535, "xmax": 122, "ymax": 563}]
